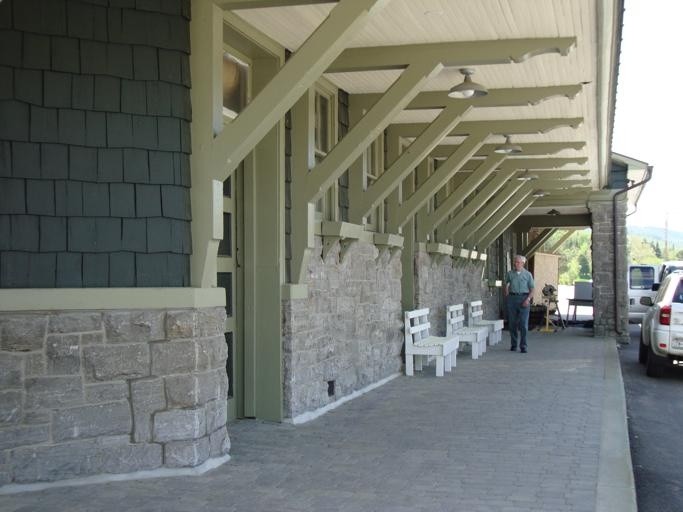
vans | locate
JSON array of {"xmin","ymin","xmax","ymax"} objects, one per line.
[{"xmin": 627, "ymin": 262, "xmax": 683, "ymax": 379}]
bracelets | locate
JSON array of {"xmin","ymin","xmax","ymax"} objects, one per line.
[{"xmin": 526, "ymin": 296, "xmax": 531, "ymax": 302}]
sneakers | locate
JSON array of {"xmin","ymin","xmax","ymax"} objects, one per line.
[
  {"xmin": 521, "ymin": 348, "xmax": 527, "ymax": 353},
  {"xmin": 511, "ymin": 346, "xmax": 517, "ymax": 351}
]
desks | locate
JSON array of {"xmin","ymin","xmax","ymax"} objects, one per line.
[{"xmin": 563, "ymin": 298, "xmax": 593, "ymax": 327}]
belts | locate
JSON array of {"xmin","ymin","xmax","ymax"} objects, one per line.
[{"xmin": 509, "ymin": 293, "xmax": 529, "ymax": 295}]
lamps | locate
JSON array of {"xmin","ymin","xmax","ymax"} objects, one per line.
[
  {"xmin": 494, "ymin": 135, "xmax": 523, "ymax": 154},
  {"xmin": 547, "ymin": 209, "xmax": 560, "ymax": 216},
  {"xmin": 447, "ymin": 68, "xmax": 488, "ymax": 100},
  {"xmin": 516, "ymin": 169, "xmax": 545, "ymax": 197}
]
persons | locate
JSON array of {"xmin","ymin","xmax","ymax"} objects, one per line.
[{"xmin": 501, "ymin": 253, "xmax": 536, "ymax": 353}]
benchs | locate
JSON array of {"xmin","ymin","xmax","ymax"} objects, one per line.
[{"xmin": 403, "ymin": 300, "xmax": 504, "ymax": 377}]
